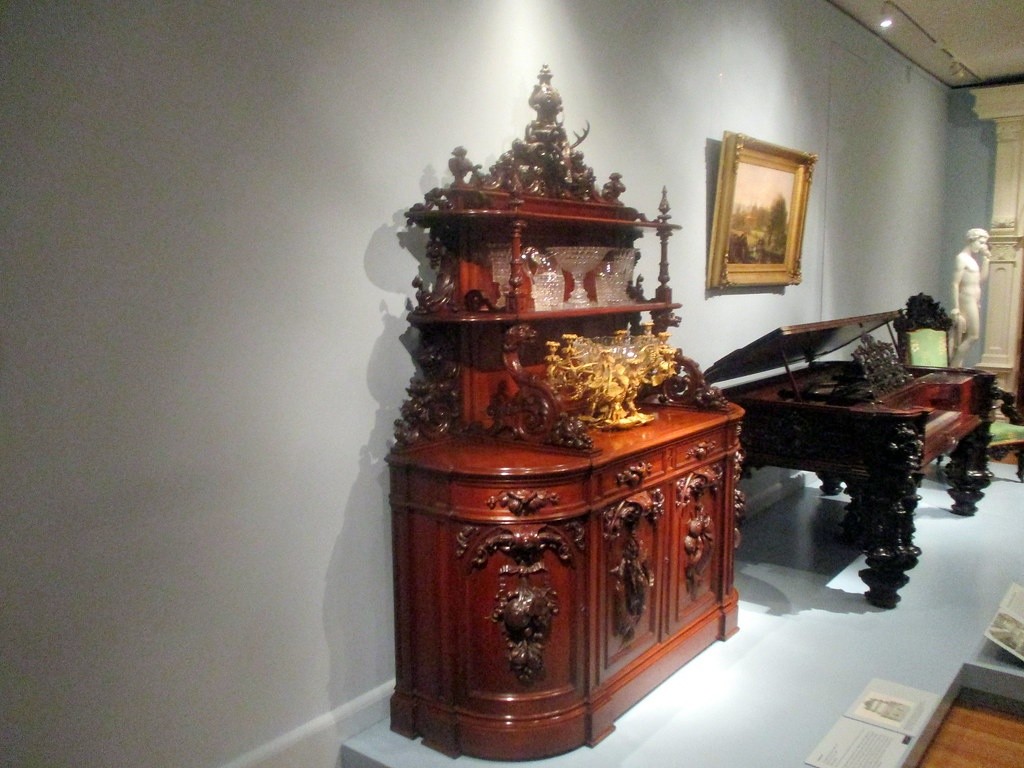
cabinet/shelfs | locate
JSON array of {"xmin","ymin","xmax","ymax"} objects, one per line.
[{"xmin": 383, "ymin": 62, "xmax": 741, "ymax": 763}]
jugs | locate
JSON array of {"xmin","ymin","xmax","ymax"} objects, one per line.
[{"xmin": 519, "ymin": 247, "xmax": 570, "ymax": 312}]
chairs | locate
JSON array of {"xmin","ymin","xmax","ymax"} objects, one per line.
[{"xmin": 893, "ymin": 291, "xmax": 1023, "ymax": 484}]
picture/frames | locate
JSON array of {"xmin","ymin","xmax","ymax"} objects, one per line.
[{"xmin": 704, "ymin": 130, "xmax": 819, "ymax": 293}]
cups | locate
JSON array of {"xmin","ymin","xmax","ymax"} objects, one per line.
[{"xmin": 602, "ymin": 247, "xmax": 636, "ymax": 305}]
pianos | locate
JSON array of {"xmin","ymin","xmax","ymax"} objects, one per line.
[{"xmin": 700, "ymin": 307, "xmax": 1001, "ymax": 611}]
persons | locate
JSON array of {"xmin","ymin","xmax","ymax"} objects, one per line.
[{"xmin": 950, "ymin": 228, "xmax": 992, "ymax": 368}]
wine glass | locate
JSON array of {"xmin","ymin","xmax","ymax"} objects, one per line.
[{"xmin": 545, "ymin": 246, "xmax": 614, "ymax": 309}]
